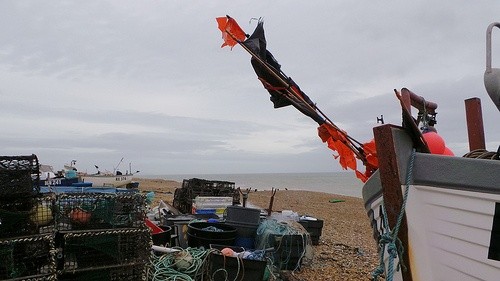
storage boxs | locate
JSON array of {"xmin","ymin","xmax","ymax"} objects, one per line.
[{"xmin": 151, "ymin": 195, "xmax": 325, "ymax": 281}]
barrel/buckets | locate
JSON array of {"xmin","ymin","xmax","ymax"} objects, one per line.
[
  {"xmin": 185, "ymin": 231, "xmax": 236, "ymax": 250},
  {"xmin": 166, "ymin": 214, "xmax": 196, "ymax": 250},
  {"xmin": 225, "ymin": 218, "xmax": 258, "ymax": 250},
  {"xmin": 225, "ymin": 205, "xmax": 261, "ymax": 225},
  {"xmin": 150, "ymin": 225, "xmax": 172, "ymax": 257},
  {"xmin": 187, "ymin": 221, "xmax": 238, "ymax": 239},
  {"xmin": 296, "ymin": 214, "xmax": 324, "ymax": 246},
  {"xmin": 267, "ymin": 232, "xmax": 311, "ymax": 272},
  {"xmin": 208, "ymin": 244, "xmax": 269, "ymax": 281}
]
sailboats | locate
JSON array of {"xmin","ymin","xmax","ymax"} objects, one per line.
[{"xmin": 78, "ymin": 172, "xmax": 134, "ymax": 188}]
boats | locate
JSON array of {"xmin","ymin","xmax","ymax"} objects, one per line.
[{"xmin": 363, "ymin": 123, "xmax": 500, "ymax": 281}]
